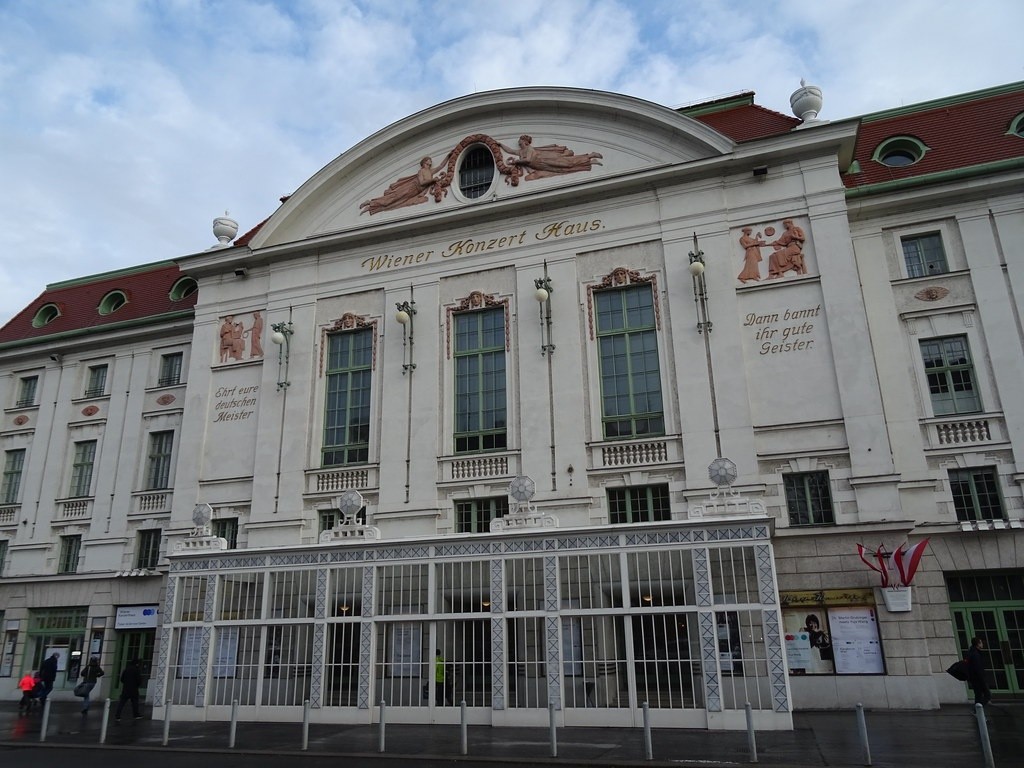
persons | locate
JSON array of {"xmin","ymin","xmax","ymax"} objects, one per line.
[
  {"xmin": 40, "ymin": 652, "xmax": 60, "ymax": 702},
  {"xmin": 244, "ymin": 311, "xmax": 264, "ymax": 358},
  {"xmin": 435, "ymin": 648, "xmax": 444, "ymax": 707},
  {"xmin": 966, "ymin": 638, "xmax": 995, "ymax": 708},
  {"xmin": 81, "ymin": 656, "xmax": 104, "ymax": 713},
  {"xmin": 611, "ymin": 267, "xmax": 630, "ymax": 287},
  {"xmin": 220, "ymin": 314, "xmax": 244, "ymax": 360},
  {"xmin": 792, "ymin": 614, "xmax": 820, "ymax": 674},
  {"xmin": 763, "ymin": 219, "xmax": 805, "ymax": 280},
  {"xmin": 737, "ymin": 228, "xmax": 767, "ymax": 282},
  {"xmin": 360, "ymin": 147, "xmax": 453, "ymax": 216},
  {"xmin": 468, "ymin": 291, "xmax": 485, "ymax": 310},
  {"xmin": 19, "ymin": 672, "xmax": 39, "ymax": 706},
  {"xmin": 343, "ymin": 313, "xmax": 354, "ymax": 328},
  {"xmin": 496, "ymin": 135, "xmax": 603, "ymax": 180},
  {"xmin": 19, "ymin": 670, "xmax": 34, "ymax": 710},
  {"xmin": 115, "ymin": 659, "xmax": 145, "ymax": 721}
]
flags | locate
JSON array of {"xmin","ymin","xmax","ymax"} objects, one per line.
[{"xmin": 854, "ymin": 534, "xmax": 932, "ymax": 587}]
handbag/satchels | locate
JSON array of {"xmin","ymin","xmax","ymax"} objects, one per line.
[
  {"xmin": 26, "ymin": 681, "xmax": 47, "ymax": 698},
  {"xmin": 74, "ymin": 682, "xmax": 89, "ymax": 698}
]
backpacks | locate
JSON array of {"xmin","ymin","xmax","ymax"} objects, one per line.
[{"xmin": 945, "ymin": 645, "xmax": 975, "ymax": 682}]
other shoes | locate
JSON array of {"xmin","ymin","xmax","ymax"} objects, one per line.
[
  {"xmin": 116, "ymin": 717, "xmax": 122, "ymax": 721},
  {"xmin": 134, "ymin": 715, "xmax": 144, "ymax": 720},
  {"xmin": 81, "ymin": 705, "xmax": 89, "ymax": 714}
]
